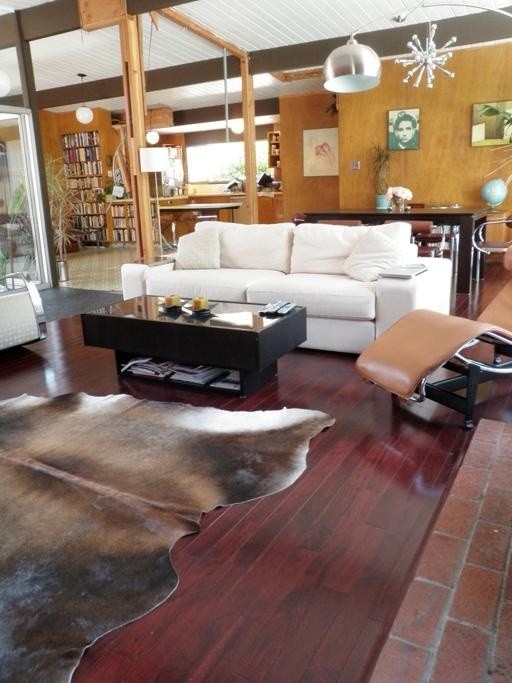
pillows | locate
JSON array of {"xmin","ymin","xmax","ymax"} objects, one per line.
[
  {"xmin": 195, "ymin": 221, "xmax": 295, "ymax": 274},
  {"xmin": 343, "ymin": 229, "xmax": 395, "ymax": 282},
  {"xmin": 289, "ymin": 223, "xmax": 413, "ymax": 279},
  {"xmin": 173, "ymin": 230, "xmax": 220, "ymax": 269}
]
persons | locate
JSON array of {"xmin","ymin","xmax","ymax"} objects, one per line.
[{"xmin": 395, "ymin": 115, "xmax": 417, "ymax": 149}]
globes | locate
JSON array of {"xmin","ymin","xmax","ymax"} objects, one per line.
[{"xmin": 482, "ymin": 179, "xmax": 508, "ymax": 214}]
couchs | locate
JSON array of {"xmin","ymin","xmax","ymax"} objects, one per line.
[{"xmin": 121, "ymin": 221, "xmax": 452, "ymax": 355}]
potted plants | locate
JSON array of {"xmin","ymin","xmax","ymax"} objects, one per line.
[
  {"xmin": 372, "ymin": 149, "xmax": 393, "ymax": 210},
  {"xmin": 44, "ymin": 160, "xmax": 78, "ymax": 280}
]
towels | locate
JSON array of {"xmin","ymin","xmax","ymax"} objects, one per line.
[{"xmin": 212, "ymin": 312, "xmax": 254, "ymax": 327}]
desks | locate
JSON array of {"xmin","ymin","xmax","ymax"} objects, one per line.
[
  {"xmin": 305, "ymin": 208, "xmax": 486, "ymax": 292},
  {"xmin": 159, "ymin": 203, "xmax": 240, "ymax": 241}
]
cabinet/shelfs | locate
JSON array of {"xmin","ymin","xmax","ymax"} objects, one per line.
[
  {"xmin": 106, "ymin": 198, "xmax": 190, "ymax": 247},
  {"xmin": 268, "ymin": 132, "xmax": 281, "ymax": 167},
  {"xmin": 58, "ymin": 107, "xmax": 118, "ymax": 247}
]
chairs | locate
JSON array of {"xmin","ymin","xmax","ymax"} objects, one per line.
[
  {"xmin": 0, "ymin": 273, "xmax": 47, "ymax": 350},
  {"xmin": 384, "ymin": 221, "xmax": 441, "ymax": 257},
  {"xmin": 416, "ymin": 223, "xmax": 459, "ymax": 277},
  {"xmin": 473, "ymin": 213, "xmax": 512, "ymax": 290},
  {"xmin": 356, "ymin": 276, "xmax": 512, "ymax": 429}
]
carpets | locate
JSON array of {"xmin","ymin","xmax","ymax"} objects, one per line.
[{"xmin": 35, "ymin": 286, "xmax": 123, "ymax": 324}]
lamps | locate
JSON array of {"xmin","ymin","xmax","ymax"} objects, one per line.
[
  {"xmin": 145, "ymin": 109, "xmax": 160, "ymax": 145},
  {"xmin": 140, "ymin": 147, "xmax": 175, "ymax": 267},
  {"xmin": 323, "ymin": 4, "xmax": 512, "ymax": 93},
  {"xmin": 76, "ymin": 75, "xmax": 92, "ymax": 124}
]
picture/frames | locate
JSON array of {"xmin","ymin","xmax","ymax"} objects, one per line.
[
  {"xmin": 469, "ymin": 101, "xmax": 512, "ymax": 146},
  {"xmin": 387, "ymin": 108, "xmax": 419, "ymax": 152}
]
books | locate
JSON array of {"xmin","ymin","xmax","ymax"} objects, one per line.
[
  {"xmin": 70, "ymin": 204, "xmax": 104, "ymax": 214},
  {"xmin": 63, "ymin": 132, "xmax": 98, "ymax": 146},
  {"xmin": 70, "ymin": 191, "xmax": 106, "ymax": 203},
  {"xmin": 113, "ymin": 230, "xmax": 137, "ymax": 240},
  {"xmin": 64, "ymin": 162, "xmax": 101, "ymax": 178},
  {"xmin": 72, "ymin": 216, "xmax": 104, "ymax": 229},
  {"xmin": 122, "ymin": 359, "xmax": 240, "ymax": 389},
  {"xmin": 79, "ymin": 230, "xmax": 105, "ymax": 241},
  {"xmin": 66, "ymin": 179, "xmax": 99, "ymax": 190},
  {"xmin": 65, "ymin": 148, "xmax": 97, "ymax": 162},
  {"xmin": 111, "ymin": 204, "xmax": 133, "ymax": 217},
  {"xmin": 113, "ymin": 219, "xmax": 136, "ymax": 228}
]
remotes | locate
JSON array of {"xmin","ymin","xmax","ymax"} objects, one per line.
[
  {"xmin": 258, "ymin": 300, "xmax": 277, "ymax": 314},
  {"xmin": 277, "ymin": 303, "xmax": 296, "ymax": 314},
  {"xmin": 266, "ymin": 300, "xmax": 289, "ymax": 315}
]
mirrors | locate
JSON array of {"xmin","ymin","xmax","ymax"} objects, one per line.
[{"xmin": 0, "ymin": 104, "xmax": 53, "ymax": 298}]
